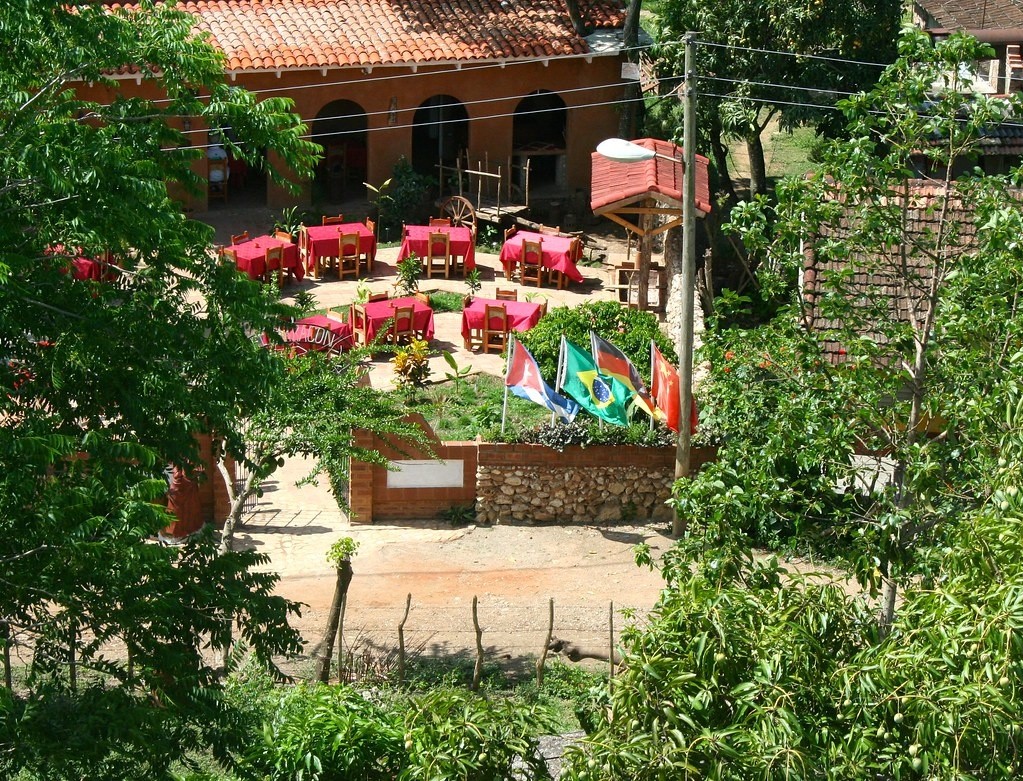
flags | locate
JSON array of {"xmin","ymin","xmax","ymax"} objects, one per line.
[
  {"xmin": 505, "ymin": 338, "xmax": 581, "ymax": 424},
  {"xmin": 592, "ymin": 335, "xmax": 662, "ymax": 420},
  {"xmin": 651, "ymin": 343, "xmax": 699, "ymax": 435},
  {"xmin": 560, "ymin": 339, "xmax": 635, "ymax": 426}
]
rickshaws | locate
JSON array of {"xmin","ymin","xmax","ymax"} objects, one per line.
[{"xmin": 433, "ymin": 148, "xmax": 586, "ymax": 245}]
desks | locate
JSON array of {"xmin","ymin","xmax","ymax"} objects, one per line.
[
  {"xmin": 45, "ymin": 245, "xmax": 117, "ymax": 296},
  {"xmin": 286, "ymin": 313, "xmax": 348, "ymax": 357},
  {"xmin": 405, "ymin": 225, "xmax": 472, "ymax": 277},
  {"xmin": 357, "ymin": 296, "xmax": 434, "ymax": 358},
  {"xmin": 462, "ymin": 297, "xmax": 541, "ymax": 351},
  {"xmin": 307, "ymin": 222, "xmax": 374, "ymax": 279},
  {"xmin": 504, "ymin": 230, "xmax": 576, "ymax": 289},
  {"xmin": 219, "ymin": 234, "xmax": 297, "ymax": 285}
]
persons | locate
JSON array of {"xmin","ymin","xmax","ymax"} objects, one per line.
[{"xmin": 208, "ymin": 133, "xmax": 247, "ymax": 192}]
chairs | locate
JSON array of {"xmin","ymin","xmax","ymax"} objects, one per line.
[{"xmin": 221, "ymin": 214, "xmax": 581, "ymax": 355}]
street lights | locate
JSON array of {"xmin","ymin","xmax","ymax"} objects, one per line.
[{"xmin": 596, "ymin": 137, "xmax": 695, "ymax": 539}]
